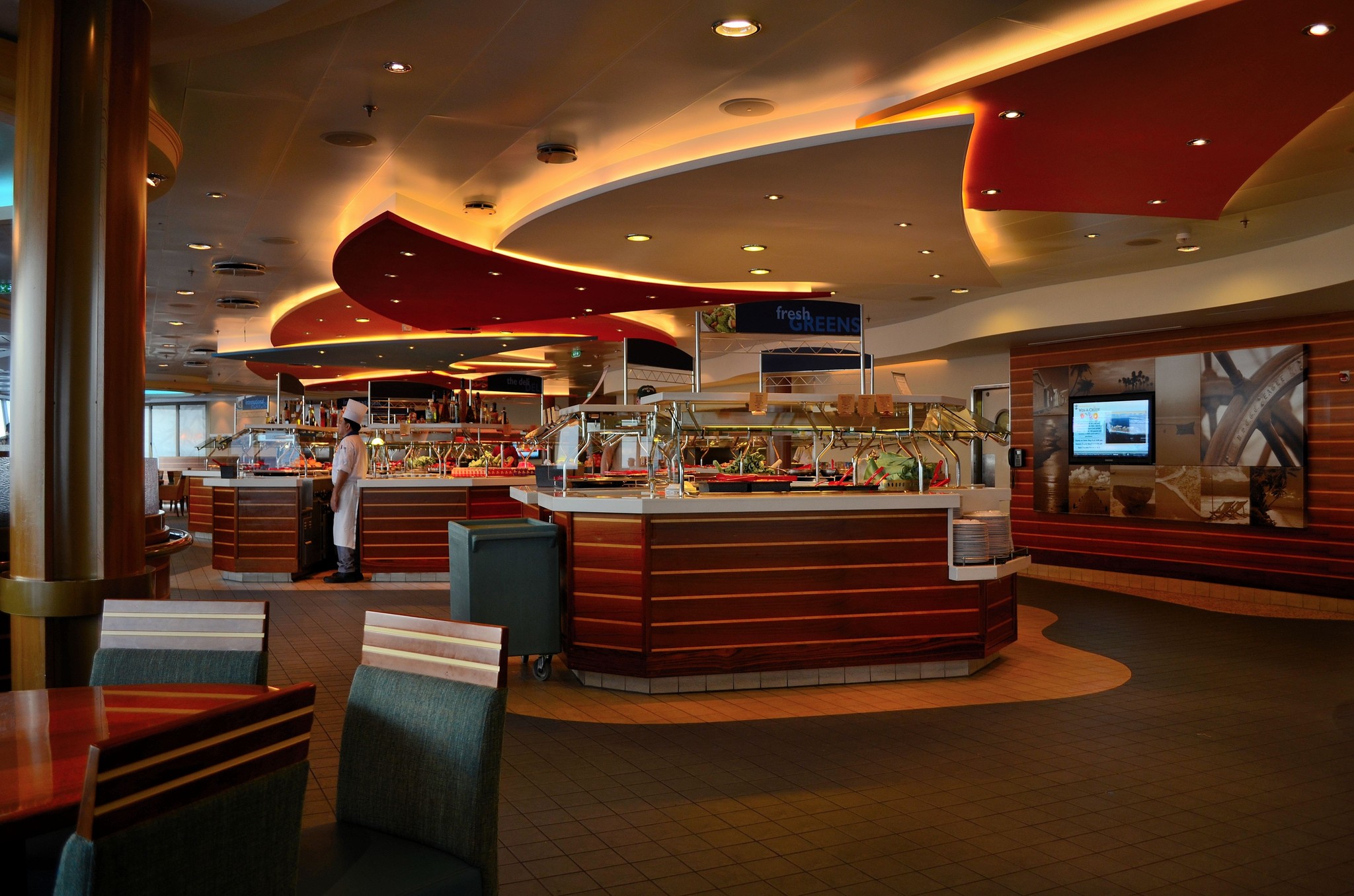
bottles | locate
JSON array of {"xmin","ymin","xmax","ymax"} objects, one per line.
[
  {"xmin": 264, "ymin": 395, "xmax": 339, "ymax": 432},
  {"xmin": 403, "ymin": 373, "xmax": 508, "ymax": 426}
]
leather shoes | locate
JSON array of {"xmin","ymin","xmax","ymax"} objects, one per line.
[{"xmin": 323, "ymin": 569, "xmax": 364, "ymax": 583}]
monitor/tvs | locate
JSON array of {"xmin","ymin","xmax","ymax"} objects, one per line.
[{"xmin": 1068, "ymin": 391, "xmax": 1155, "ymax": 466}]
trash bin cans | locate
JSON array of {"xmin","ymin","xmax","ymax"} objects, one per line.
[{"xmin": 446, "ymin": 517, "xmax": 560, "ymax": 680}]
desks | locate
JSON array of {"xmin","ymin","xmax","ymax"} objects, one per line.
[{"xmin": 1, "ymin": 686, "xmax": 284, "ymax": 896}]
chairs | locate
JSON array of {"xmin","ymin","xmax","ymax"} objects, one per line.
[
  {"xmin": 158, "ymin": 470, "xmax": 164, "ymax": 509},
  {"xmin": 293, "ymin": 610, "xmax": 506, "ymax": 894},
  {"xmin": 158, "ymin": 472, "xmax": 186, "ymax": 517},
  {"xmin": 167, "ymin": 471, "xmax": 188, "ymax": 512},
  {"xmin": 51, "ymin": 679, "xmax": 315, "ymax": 896},
  {"xmin": 90, "ymin": 597, "xmax": 269, "ymax": 685}
]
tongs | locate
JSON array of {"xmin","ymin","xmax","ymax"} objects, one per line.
[{"xmin": 707, "ymin": 473, "xmax": 757, "ymax": 483}]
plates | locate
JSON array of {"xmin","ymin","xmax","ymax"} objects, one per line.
[{"xmin": 949, "ymin": 492, "xmax": 1015, "ymax": 567}]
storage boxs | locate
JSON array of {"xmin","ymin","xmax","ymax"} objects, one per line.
[
  {"xmin": 220, "ymin": 466, "xmax": 237, "ymax": 478},
  {"xmin": 535, "ymin": 464, "xmax": 564, "ymax": 488},
  {"xmin": 695, "ymin": 481, "xmax": 792, "ymax": 493}
]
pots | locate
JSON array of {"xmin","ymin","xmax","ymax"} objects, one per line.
[
  {"xmin": 766, "ymin": 464, "xmax": 819, "ymax": 480},
  {"xmin": 820, "ymin": 467, "xmax": 853, "ymax": 481}
]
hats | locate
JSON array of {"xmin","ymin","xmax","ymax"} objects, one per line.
[{"xmin": 342, "ymin": 398, "xmax": 368, "ymax": 425}]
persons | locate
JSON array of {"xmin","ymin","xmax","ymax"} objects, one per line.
[
  {"xmin": 321, "ymin": 408, "xmax": 369, "ymax": 585},
  {"xmin": 627, "ymin": 457, "xmax": 636, "ymax": 470},
  {"xmin": 599, "ymin": 384, "xmax": 671, "ymax": 477},
  {"xmin": 0, "ymin": 421, "xmax": 10, "ymax": 445}
]
